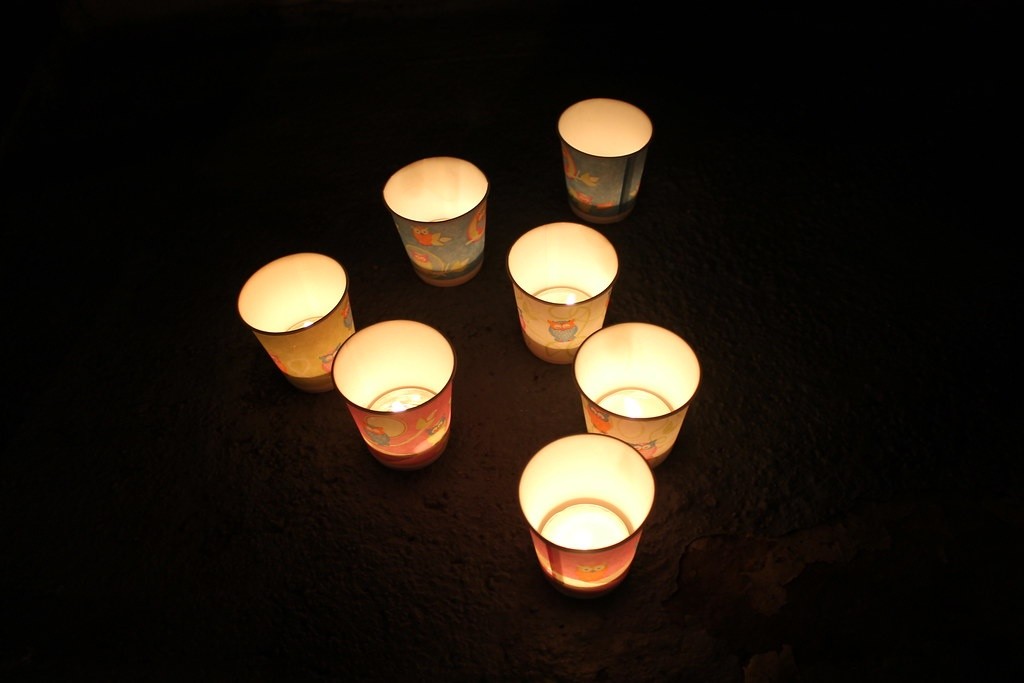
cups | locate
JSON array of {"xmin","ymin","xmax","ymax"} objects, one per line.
[
  {"xmin": 332, "ymin": 321, "xmax": 456, "ymax": 466},
  {"xmin": 518, "ymin": 435, "xmax": 655, "ymax": 591},
  {"xmin": 382, "ymin": 158, "xmax": 490, "ymax": 288},
  {"xmin": 576, "ymin": 321, "xmax": 702, "ymax": 467},
  {"xmin": 508, "ymin": 223, "xmax": 618, "ymax": 365},
  {"xmin": 239, "ymin": 252, "xmax": 355, "ymax": 390},
  {"xmin": 558, "ymin": 99, "xmax": 652, "ymax": 222}
]
